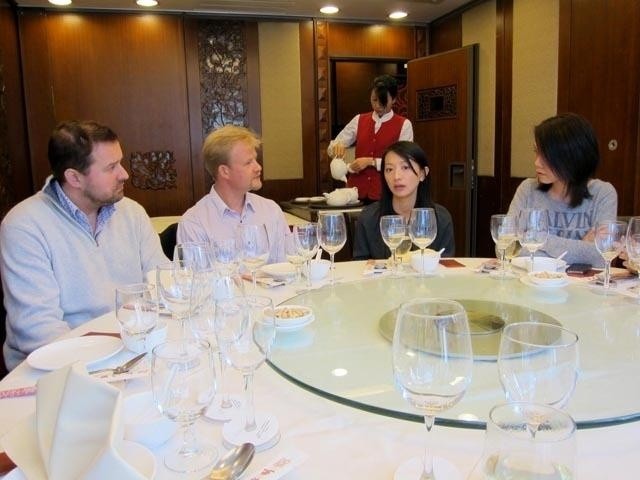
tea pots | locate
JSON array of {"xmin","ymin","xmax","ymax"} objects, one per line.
[
  {"xmin": 323, "ymin": 189, "xmax": 351, "ymax": 206},
  {"xmin": 329, "ymin": 153, "xmax": 349, "ymax": 183},
  {"xmin": 338, "ymin": 187, "xmax": 359, "ymax": 202}
]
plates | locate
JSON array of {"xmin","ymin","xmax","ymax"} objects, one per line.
[
  {"xmin": 26, "ymin": 335, "xmax": 124, "ymax": 371},
  {"xmin": 262, "ymin": 305, "xmax": 313, "ymax": 327},
  {"xmin": 520, "ymin": 276, "xmax": 570, "ymax": 288},
  {"xmin": 528, "ymin": 271, "xmax": 567, "ymax": 286},
  {"xmin": 257, "ymin": 314, "xmax": 315, "ymax": 333},
  {"xmin": 308, "ymin": 196, "xmax": 374, "ymax": 210},
  {"xmin": 260, "ymin": 262, "xmax": 298, "ymax": 278},
  {"xmin": 289, "ymin": 195, "xmax": 325, "ymax": 205},
  {"xmin": 513, "ymin": 254, "xmax": 566, "ymax": 272}
]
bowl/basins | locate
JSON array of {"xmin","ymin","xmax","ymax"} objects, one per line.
[
  {"xmin": 121, "ymin": 319, "xmax": 168, "ymax": 354},
  {"xmin": 409, "ymin": 252, "xmax": 440, "ymax": 273},
  {"xmin": 303, "ymin": 259, "xmax": 331, "ymax": 281}
]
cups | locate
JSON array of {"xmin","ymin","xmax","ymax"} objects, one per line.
[
  {"xmin": 237, "ymin": 224, "xmax": 271, "ymax": 294},
  {"xmin": 215, "ymin": 296, "xmax": 279, "ymax": 446},
  {"xmin": 189, "ymin": 268, "xmax": 248, "ymax": 393},
  {"xmin": 294, "ymin": 223, "xmax": 321, "ymax": 295},
  {"xmin": 518, "ymin": 209, "xmax": 549, "ymax": 276},
  {"xmin": 151, "ymin": 338, "xmax": 217, "ymax": 472},
  {"xmin": 497, "ymin": 322, "xmax": 579, "ymax": 439},
  {"xmin": 394, "ymin": 299, "xmax": 473, "ymax": 479},
  {"xmin": 316, "ymin": 212, "xmax": 347, "ymax": 286},
  {"xmin": 285, "ymin": 232, "xmax": 309, "ymax": 286},
  {"xmin": 389, "ymin": 226, "xmax": 412, "ymax": 260},
  {"xmin": 380, "ymin": 216, "xmax": 407, "ymax": 279},
  {"xmin": 157, "ymin": 260, "xmax": 201, "ymax": 341},
  {"xmin": 491, "ymin": 214, "xmax": 516, "ymax": 281},
  {"xmin": 116, "ymin": 283, "xmax": 160, "ymax": 379},
  {"xmin": 211, "ymin": 237, "xmax": 242, "ymax": 298},
  {"xmin": 592, "ymin": 220, "xmax": 626, "ymax": 295},
  {"xmin": 409, "ymin": 208, "xmax": 437, "ymax": 279},
  {"xmin": 482, "ymin": 403, "xmax": 576, "ymax": 479},
  {"xmin": 625, "ymin": 217, "xmax": 640, "ymax": 304},
  {"xmin": 174, "ymin": 242, "xmax": 212, "ymax": 287}
]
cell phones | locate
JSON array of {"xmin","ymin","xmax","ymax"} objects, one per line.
[{"xmin": 566, "ymin": 262, "xmax": 592, "ymax": 275}]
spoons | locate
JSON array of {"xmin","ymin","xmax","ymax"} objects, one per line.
[{"xmin": 197, "ymin": 443, "xmax": 255, "ymax": 480}]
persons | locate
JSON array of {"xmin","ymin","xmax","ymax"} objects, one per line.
[
  {"xmin": 176, "ymin": 124, "xmax": 302, "ymax": 271},
  {"xmin": 0, "ymin": 119, "xmax": 172, "ymax": 371},
  {"xmin": 352, "ymin": 140, "xmax": 456, "ymax": 261},
  {"xmin": 494, "ymin": 112, "xmax": 618, "ymax": 268},
  {"xmin": 327, "ymin": 76, "xmax": 414, "ymax": 205}
]
